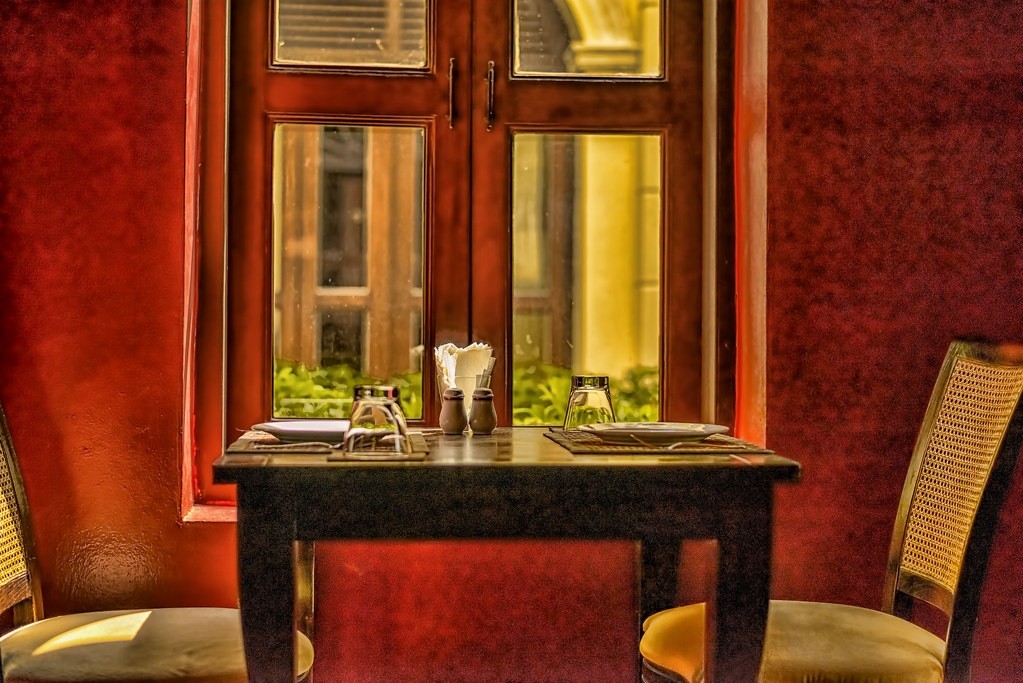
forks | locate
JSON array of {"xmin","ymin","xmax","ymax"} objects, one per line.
[
  {"xmin": 630, "ymin": 434, "xmax": 746, "ymax": 450},
  {"xmin": 255, "ymin": 435, "xmax": 365, "ymax": 448}
]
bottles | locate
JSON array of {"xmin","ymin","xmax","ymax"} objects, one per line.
[
  {"xmin": 439, "ymin": 388, "xmax": 466, "ymax": 434},
  {"xmin": 470, "ymin": 389, "xmax": 498, "ymax": 435}
]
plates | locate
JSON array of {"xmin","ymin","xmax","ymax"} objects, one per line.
[
  {"xmin": 577, "ymin": 421, "xmax": 731, "ymax": 444},
  {"xmin": 253, "ymin": 421, "xmax": 351, "ymax": 443}
]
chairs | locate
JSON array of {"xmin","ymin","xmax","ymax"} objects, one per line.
[
  {"xmin": 0, "ymin": 402, "xmax": 316, "ymax": 683},
  {"xmin": 638, "ymin": 342, "xmax": 1023, "ymax": 683}
]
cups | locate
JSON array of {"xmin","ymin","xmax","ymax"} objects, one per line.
[
  {"xmin": 345, "ymin": 384, "xmax": 412, "ymax": 455},
  {"xmin": 564, "ymin": 377, "xmax": 615, "ymax": 432}
]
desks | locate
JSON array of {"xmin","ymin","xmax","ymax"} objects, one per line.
[{"xmin": 212, "ymin": 425, "xmax": 801, "ymax": 683}]
